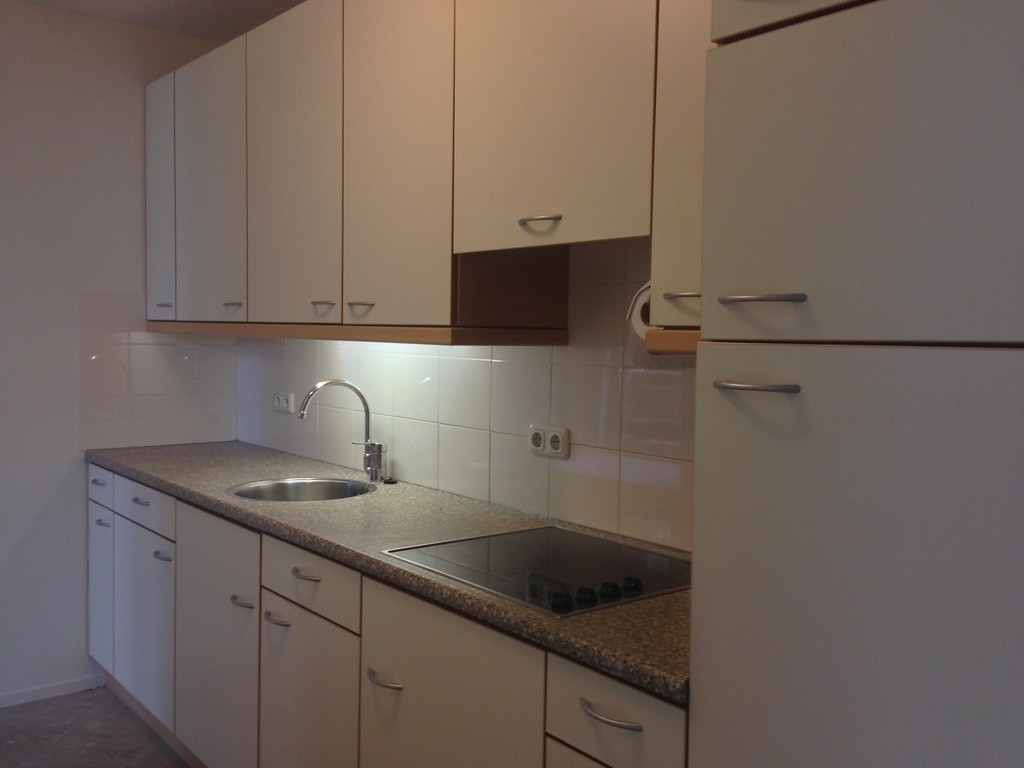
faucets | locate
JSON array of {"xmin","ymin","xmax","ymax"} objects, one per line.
[{"xmin": 297, "ymin": 378, "xmax": 371, "ymax": 473}]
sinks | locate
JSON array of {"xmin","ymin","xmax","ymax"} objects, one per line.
[{"xmin": 225, "ymin": 477, "xmax": 377, "ymax": 503}]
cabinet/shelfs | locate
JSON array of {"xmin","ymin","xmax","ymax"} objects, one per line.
[
  {"xmin": 648, "ymin": 0, "xmax": 1024, "ymax": 768},
  {"xmin": 88, "ymin": 460, "xmax": 687, "ymax": 768},
  {"xmin": 146, "ymin": 0, "xmax": 664, "ymax": 346}
]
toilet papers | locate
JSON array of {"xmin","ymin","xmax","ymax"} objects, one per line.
[{"xmin": 626, "ymin": 277, "xmax": 666, "ymax": 340}]
